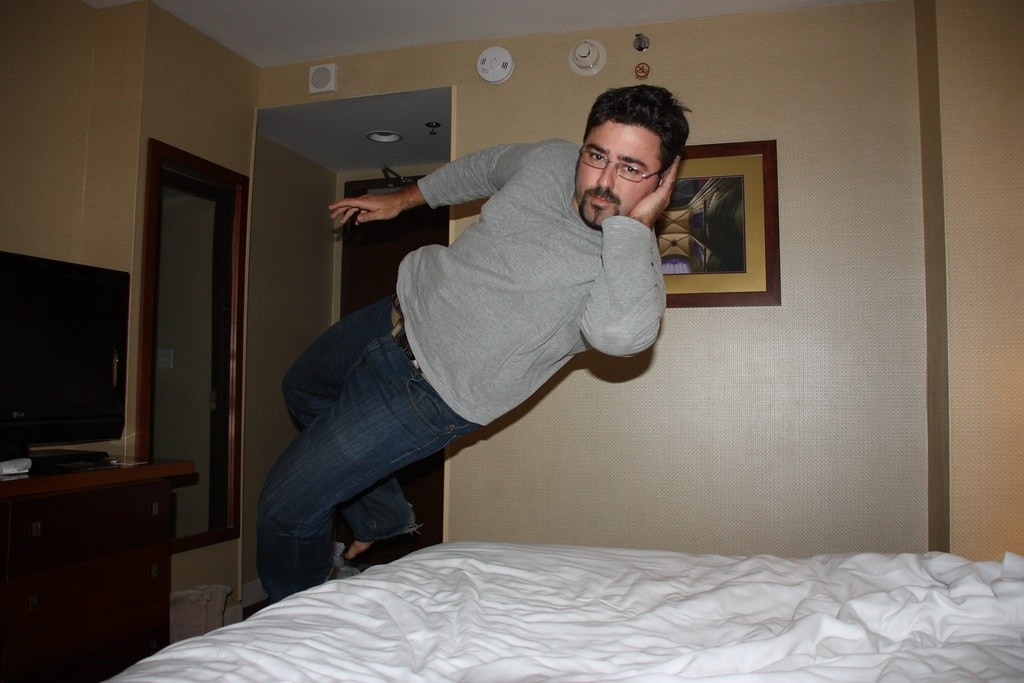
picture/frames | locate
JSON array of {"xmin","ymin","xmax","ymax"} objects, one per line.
[{"xmin": 654, "ymin": 139, "xmax": 782, "ymax": 308}]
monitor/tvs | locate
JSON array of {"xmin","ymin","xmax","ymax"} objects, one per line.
[{"xmin": 0, "ymin": 250, "xmax": 131, "ymax": 479}]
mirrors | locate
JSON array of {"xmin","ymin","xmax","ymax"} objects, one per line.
[{"xmin": 133, "ymin": 137, "xmax": 241, "ymax": 555}]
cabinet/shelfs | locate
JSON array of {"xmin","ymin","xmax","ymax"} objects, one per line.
[{"xmin": 0, "ymin": 455, "xmax": 199, "ymax": 683}]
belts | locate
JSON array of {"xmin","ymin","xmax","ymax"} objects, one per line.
[{"xmin": 391, "ymin": 295, "xmax": 419, "ymax": 369}]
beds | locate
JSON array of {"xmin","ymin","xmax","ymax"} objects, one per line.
[{"xmin": 104, "ymin": 542, "xmax": 1024, "ymax": 683}]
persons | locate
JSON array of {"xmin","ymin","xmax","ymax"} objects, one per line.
[{"xmin": 254, "ymin": 83, "xmax": 693, "ymax": 605}]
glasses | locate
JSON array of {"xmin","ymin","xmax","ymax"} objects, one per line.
[{"xmin": 577, "ymin": 144, "xmax": 667, "ymax": 183}]
